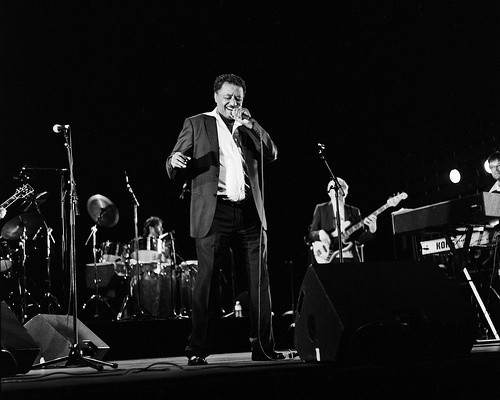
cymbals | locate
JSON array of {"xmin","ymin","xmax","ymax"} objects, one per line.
[
  {"xmin": 86, "ymin": 194, "xmax": 119, "ymax": 228},
  {"xmin": 94, "ymin": 242, "xmax": 111, "ymax": 249},
  {"xmin": 1, "ymin": 211, "xmax": 41, "ymax": 241}
]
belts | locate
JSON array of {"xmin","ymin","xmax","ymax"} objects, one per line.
[{"xmin": 217, "ymin": 198, "xmax": 254, "ymax": 208}]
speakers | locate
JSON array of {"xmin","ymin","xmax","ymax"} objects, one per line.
[
  {"xmin": 293, "ymin": 262, "xmax": 478, "ymax": 364},
  {"xmin": 23, "ymin": 313, "xmax": 111, "ymax": 368},
  {"xmin": 0, "ymin": 300, "xmax": 42, "ymax": 377}
]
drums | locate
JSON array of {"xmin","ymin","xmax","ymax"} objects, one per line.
[
  {"xmin": 130, "ymin": 234, "xmax": 174, "ymax": 267},
  {"xmin": 98, "ymin": 241, "xmax": 130, "ymax": 262},
  {"xmin": 176, "ymin": 258, "xmax": 200, "ymax": 318},
  {"xmin": 0, "ymin": 236, "xmax": 14, "ymax": 273}
]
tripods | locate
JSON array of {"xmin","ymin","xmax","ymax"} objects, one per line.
[{"xmin": 2, "ymin": 185, "xmax": 173, "ymax": 371}]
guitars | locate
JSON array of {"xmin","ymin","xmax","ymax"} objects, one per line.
[
  {"xmin": 311, "ymin": 189, "xmax": 409, "ymax": 264},
  {"xmin": 0, "ymin": 183, "xmax": 34, "ymax": 208}
]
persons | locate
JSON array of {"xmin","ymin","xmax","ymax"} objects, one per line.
[
  {"xmin": 166, "ymin": 73, "xmax": 285, "ymax": 365},
  {"xmin": 304, "ymin": 177, "xmax": 377, "ymax": 263},
  {"xmin": 488, "ymin": 151, "xmax": 500, "ymax": 194},
  {"xmin": 128, "ymin": 217, "xmax": 176, "ymax": 311}
]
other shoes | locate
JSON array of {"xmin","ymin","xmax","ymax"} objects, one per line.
[
  {"xmin": 252, "ymin": 351, "xmax": 285, "ymax": 360},
  {"xmin": 188, "ymin": 356, "xmax": 208, "ymax": 365}
]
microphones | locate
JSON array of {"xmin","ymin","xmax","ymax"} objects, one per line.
[
  {"xmin": 125, "ymin": 175, "xmax": 129, "ymax": 187},
  {"xmin": 179, "ymin": 183, "xmax": 187, "ymax": 199},
  {"xmin": 53, "ymin": 124, "xmax": 69, "ymax": 133},
  {"xmin": 14, "ymin": 165, "xmax": 27, "ymax": 180}
]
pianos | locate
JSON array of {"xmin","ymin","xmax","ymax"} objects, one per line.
[{"xmin": 391, "ymin": 190, "xmax": 500, "ymax": 341}]
